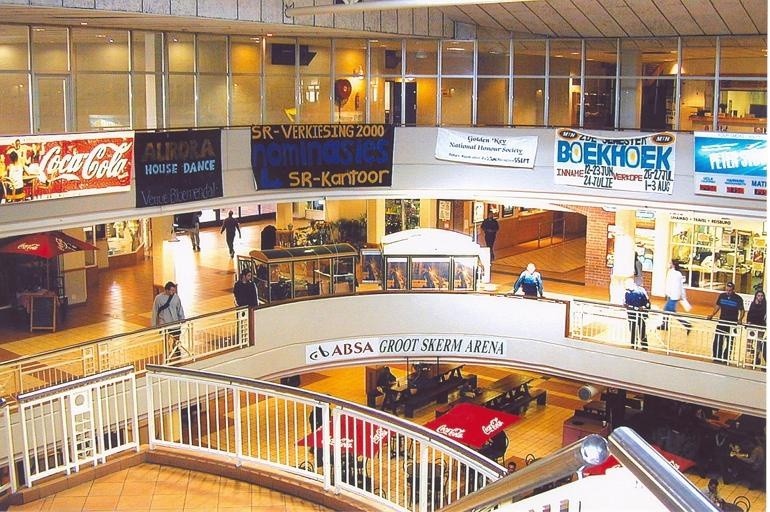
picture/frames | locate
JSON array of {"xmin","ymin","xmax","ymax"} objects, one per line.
[
  {"xmin": 604, "ymin": 223, "xmax": 655, "ymax": 273},
  {"xmin": 472, "ymin": 201, "xmax": 514, "ymax": 223}
]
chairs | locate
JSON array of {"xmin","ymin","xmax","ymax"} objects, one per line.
[
  {"xmin": 402, "ymin": 454, "xmax": 451, "ymax": 506},
  {"xmin": 733, "ymin": 495, "xmax": 751, "ymax": 511},
  {"xmin": 524, "ymin": 452, "xmax": 536, "ymax": 467},
  {"xmin": 299, "ymin": 444, "xmax": 387, "ymax": 497}
]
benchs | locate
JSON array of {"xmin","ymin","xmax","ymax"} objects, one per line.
[
  {"xmin": 575, "ymin": 410, "xmax": 606, "ymax": 423},
  {"xmin": 498, "ymin": 388, "xmax": 547, "ymax": 413},
  {"xmin": 435, "ymin": 387, "xmax": 483, "ymax": 418},
  {"xmin": 402, "ymin": 374, "xmax": 477, "ymax": 418},
  {"xmin": 600, "ymin": 392, "xmax": 641, "ymax": 410}
]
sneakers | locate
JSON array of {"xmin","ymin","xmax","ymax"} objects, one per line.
[
  {"xmin": 686, "ymin": 325, "xmax": 691, "ymax": 336},
  {"xmin": 230, "ymin": 249, "xmax": 234, "ymax": 258},
  {"xmin": 193, "ymin": 244, "xmax": 200, "ymax": 251},
  {"xmin": 657, "ymin": 325, "xmax": 668, "ymax": 330}
]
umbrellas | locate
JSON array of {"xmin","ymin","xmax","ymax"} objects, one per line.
[
  {"xmin": 297, "ymin": 414, "xmax": 397, "ymax": 458},
  {"xmin": 423, "ymin": 401, "xmax": 521, "ymax": 449},
  {"xmin": 0, "ymin": 231, "xmax": 99, "ymax": 289}
]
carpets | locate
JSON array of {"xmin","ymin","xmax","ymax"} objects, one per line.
[{"xmin": 438, "ymin": 455, "xmax": 532, "ymax": 506}]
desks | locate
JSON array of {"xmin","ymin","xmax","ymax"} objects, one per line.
[
  {"xmin": 464, "ymin": 372, "xmax": 534, "ymax": 407},
  {"xmin": 583, "ymin": 399, "xmax": 642, "ymax": 423},
  {"xmin": 713, "ymin": 498, "xmax": 745, "ymax": 512},
  {"xmin": 382, "ymin": 365, "xmax": 464, "ymax": 418}
]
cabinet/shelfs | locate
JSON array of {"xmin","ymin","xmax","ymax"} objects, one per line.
[{"xmin": 562, "ymin": 416, "xmax": 612, "ymax": 448}]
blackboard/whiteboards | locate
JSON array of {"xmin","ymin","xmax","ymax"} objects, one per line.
[{"xmin": 30, "ymin": 295, "xmax": 57, "ymax": 329}]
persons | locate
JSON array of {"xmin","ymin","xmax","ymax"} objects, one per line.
[
  {"xmin": 376, "ymin": 366, "xmax": 399, "ymax": 416},
  {"xmin": 151, "ymin": 281, "xmax": 187, "ymax": 363},
  {"xmin": 0, "ymin": 138, "xmax": 48, "ymax": 203},
  {"xmin": 634, "ymin": 251, "xmax": 645, "ymax": 288},
  {"xmin": 220, "ymin": 210, "xmax": 241, "ymax": 259},
  {"xmin": 308, "ymin": 393, "xmax": 332, "ymax": 455},
  {"xmin": 508, "ymin": 462, "xmax": 517, "ymax": 475},
  {"xmin": 706, "ymin": 281, "xmax": 745, "ymax": 366},
  {"xmin": 745, "ymin": 289, "xmax": 766, "ymax": 373},
  {"xmin": 700, "ymin": 251, "xmax": 722, "ymax": 280},
  {"xmin": 481, "ymin": 210, "xmax": 500, "ymax": 261},
  {"xmin": 189, "ymin": 211, "xmax": 202, "ymax": 251},
  {"xmin": 687, "ymin": 405, "xmax": 766, "ymax": 507},
  {"xmin": 656, "ymin": 258, "xmax": 693, "ymax": 336},
  {"xmin": 624, "ymin": 277, "xmax": 651, "ymax": 353},
  {"xmin": 232, "ymin": 268, "xmax": 260, "ymax": 312},
  {"xmin": 476, "ymin": 429, "xmax": 508, "ymax": 460},
  {"xmin": 506, "ymin": 261, "xmax": 546, "ymax": 300}
]
